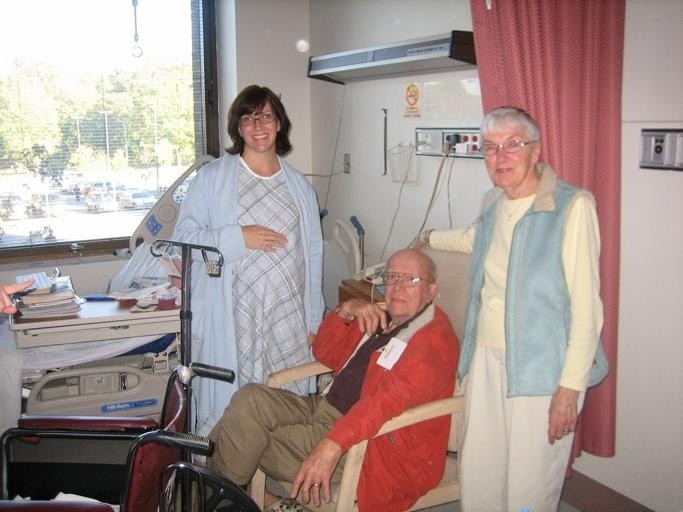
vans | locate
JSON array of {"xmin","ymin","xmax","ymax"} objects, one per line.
[{"xmin": 91, "ymin": 180, "xmax": 113, "ymax": 191}]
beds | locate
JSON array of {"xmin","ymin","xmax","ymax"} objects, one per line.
[{"xmin": 0, "ymin": 153, "xmax": 365, "ymax": 433}]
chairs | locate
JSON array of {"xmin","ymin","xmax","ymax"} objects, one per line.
[{"xmin": 247, "ymin": 244, "xmax": 472, "ymax": 512}]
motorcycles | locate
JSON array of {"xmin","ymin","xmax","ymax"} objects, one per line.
[
  {"xmin": 0, "ymin": 197, "xmax": 15, "ymax": 221},
  {"xmin": 25, "ymin": 202, "xmax": 42, "ymax": 216}
]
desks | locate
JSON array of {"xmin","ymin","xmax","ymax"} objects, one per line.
[{"xmin": 7, "ymin": 297, "xmax": 182, "ymax": 350}]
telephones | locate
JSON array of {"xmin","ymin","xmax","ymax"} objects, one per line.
[{"xmin": 361, "ymin": 261, "xmax": 389, "ymax": 284}]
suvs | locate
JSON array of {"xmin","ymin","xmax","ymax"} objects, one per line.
[{"xmin": 86, "ymin": 191, "xmax": 117, "ymax": 213}]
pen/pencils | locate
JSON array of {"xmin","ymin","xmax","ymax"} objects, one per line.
[{"xmin": 84, "ymin": 296, "xmax": 116, "ymax": 301}]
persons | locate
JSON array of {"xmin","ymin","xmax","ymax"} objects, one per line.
[
  {"xmin": 421, "ymin": 106, "xmax": 609, "ymax": 512},
  {"xmin": 206, "ymin": 249, "xmax": 460, "ymax": 512},
  {"xmin": 170, "ymin": 85, "xmax": 325, "ymax": 508},
  {"xmin": 0, "ymin": 279, "xmax": 35, "ymax": 314}
]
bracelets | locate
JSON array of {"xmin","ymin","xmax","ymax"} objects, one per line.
[
  {"xmin": 424, "ymin": 229, "xmax": 435, "ymax": 246},
  {"xmin": 312, "ymin": 483, "xmax": 320, "ymax": 488}
]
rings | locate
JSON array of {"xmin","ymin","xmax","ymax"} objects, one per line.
[
  {"xmin": 265, "ymin": 240, "xmax": 272, "ymax": 246},
  {"xmin": 564, "ymin": 428, "xmax": 569, "ymax": 432}
]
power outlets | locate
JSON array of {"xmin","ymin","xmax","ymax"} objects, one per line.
[{"xmin": 414, "ymin": 126, "xmax": 488, "ymax": 158}]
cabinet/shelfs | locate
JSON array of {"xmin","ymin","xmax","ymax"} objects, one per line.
[{"xmin": 336, "ymin": 278, "xmax": 386, "ymax": 306}]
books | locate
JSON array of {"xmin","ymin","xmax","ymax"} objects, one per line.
[{"xmin": 12, "ymin": 272, "xmax": 86, "ymax": 318}]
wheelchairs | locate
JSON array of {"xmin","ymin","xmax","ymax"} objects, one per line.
[{"xmin": 0, "ymin": 362, "xmax": 261, "ymax": 512}]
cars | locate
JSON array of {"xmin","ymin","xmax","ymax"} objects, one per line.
[{"xmin": 120, "ymin": 190, "xmax": 156, "ymax": 210}]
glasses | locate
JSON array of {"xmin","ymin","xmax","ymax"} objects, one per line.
[
  {"xmin": 240, "ymin": 114, "xmax": 275, "ymax": 127},
  {"xmin": 479, "ymin": 141, "xmax": 535, "ymax": 155},
  {"xmin": 382, "ymin": 272, "xmax": 431, "ymax": 287}
]
